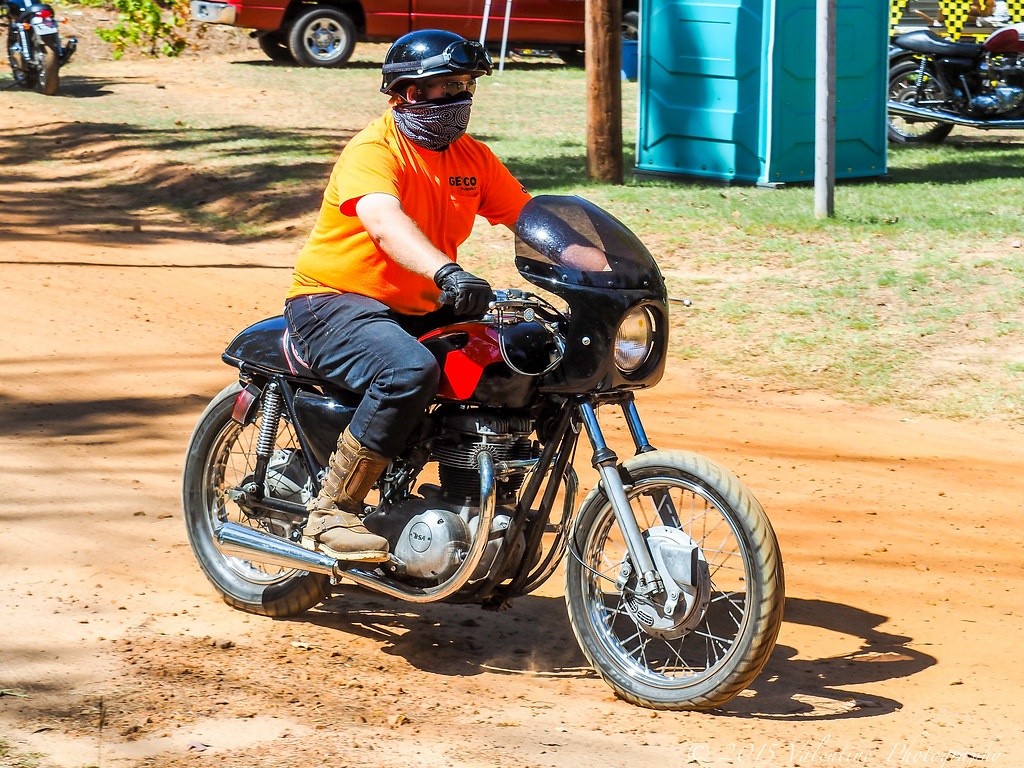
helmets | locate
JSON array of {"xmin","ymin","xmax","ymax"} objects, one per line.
[{"xmin": 380, "ymin": 30, "xmax": 487, "ymax": 96}]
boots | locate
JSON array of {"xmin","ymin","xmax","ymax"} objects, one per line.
[{"xmin": 303, "ymin": 423, "xmax": 391, "ymax": 563}]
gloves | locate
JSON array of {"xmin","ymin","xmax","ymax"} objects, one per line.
[{"xmin": 434, "ymin": 262, "xmax": 496, "ymax": 322}]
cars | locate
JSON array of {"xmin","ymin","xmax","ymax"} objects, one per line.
[{"xmin": 186, "ymin": 0, "xmax": 585, "ymax": 70}]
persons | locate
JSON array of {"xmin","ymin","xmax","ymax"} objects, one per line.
[
  {"xmin": 6, "ymin": 0, "xmax": 42, "ymax": 69},
  {"xmin": 909, "ymin": 0, "xmax": 1009, "ymax": 29},
  {"xmin": 283, "ymin": 31, "xmax": 648, "ymax": 563}
]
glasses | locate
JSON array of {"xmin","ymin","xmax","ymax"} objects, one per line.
[
  {"xmin": 421, "ymin": 79, "xmax": 480, "ymax": 95},
  {"xmin": 448, "ymin": 41, "xmax": 493, "ymax": 76}
]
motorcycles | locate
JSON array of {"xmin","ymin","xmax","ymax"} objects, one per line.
[
  {"xmin": 0, "ymin": 0, "xmax": 79, "ymax": 97},
  {"xmin": 884, "ymin": 28, "xmax": 1024, "ymax": 146},
  {"xmin": 182, "ymin": 193, "xmax": 785, "ymax": 713}
]
